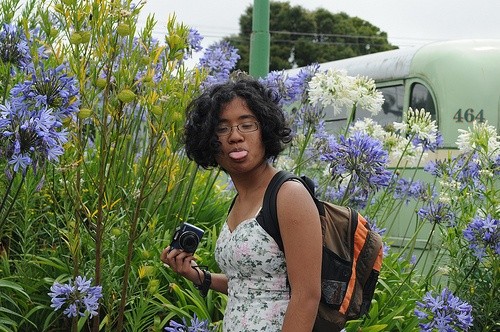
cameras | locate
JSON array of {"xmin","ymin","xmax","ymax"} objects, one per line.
[{"xmin": 170, "ymin": 222, "xmax": 204, "ymax": 257}]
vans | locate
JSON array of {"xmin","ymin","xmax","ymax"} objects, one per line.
[{"xmin": 249, "ymin": 38, "xmax": 500, "ymax": 302}]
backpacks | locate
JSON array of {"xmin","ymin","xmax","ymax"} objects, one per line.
[{"xmin": 226, "ymin": 169, "xmax": 384, "ymax": 332}]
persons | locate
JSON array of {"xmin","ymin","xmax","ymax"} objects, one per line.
[{"xmin": 159, "ymin": 79, "xmax": 324, "ymax": 332}]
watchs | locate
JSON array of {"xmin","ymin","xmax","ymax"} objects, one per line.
[{"xmin": 192, "ymin": 269, "xmax": 211, "ymax": 297}]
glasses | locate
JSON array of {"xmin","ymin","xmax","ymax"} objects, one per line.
[{"xmin": 215, "ymin": 121, "xmax": 261, "ymax": 135}]
